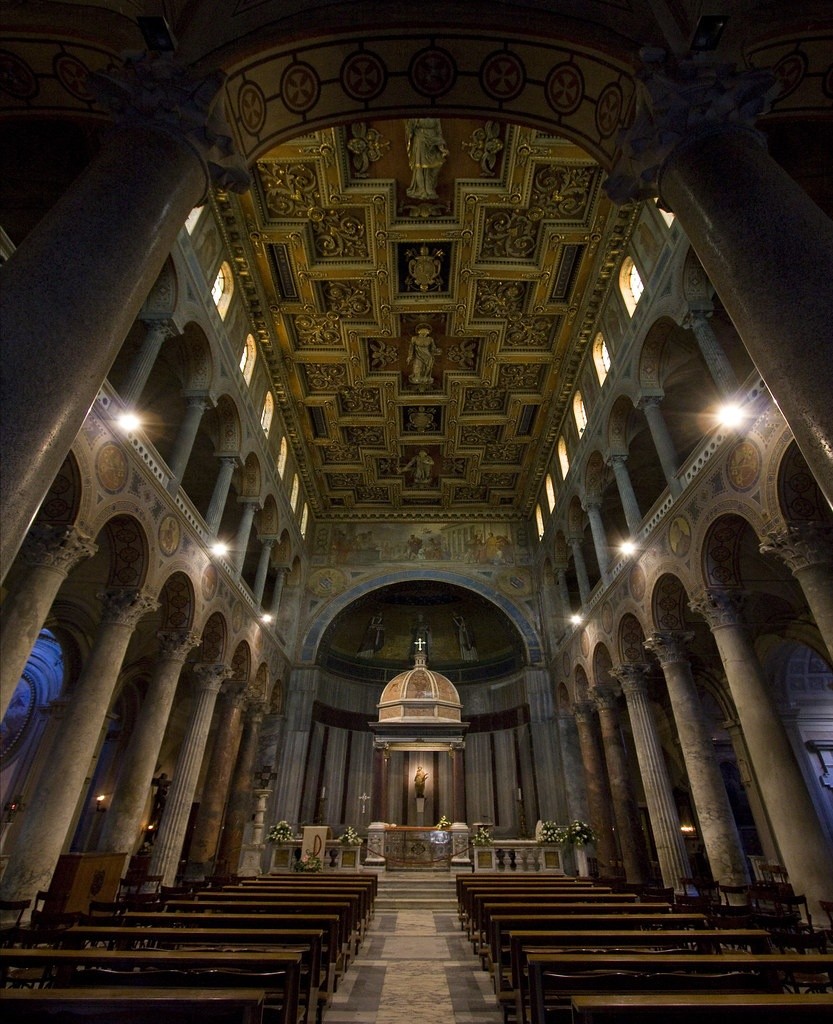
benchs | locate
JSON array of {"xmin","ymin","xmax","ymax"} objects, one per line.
[
  {"xmin": 569, "ymin": 991, "xmax": 833, "ymax": 1024},
  {"xmin": 64, "ymin": 925, "xmax": 332, "ymax": 1024},
  {"xmin": 122, "ymin": 868, "xmax": 708, "ymax": 997},
  {"xmin": 0, "ymin": 988, "xmax": 265, "ymax": 1024},
  {"xmin": 0, "ymin": 948, "xmax": 306, "ymax": 1023},
  {"xmin": 526, "ymin": 953, "xmax": 833, "ymax": 1024},
  {"xmin": 495, "ymin": 930, "xmax": 771, "ymax": 1024}
]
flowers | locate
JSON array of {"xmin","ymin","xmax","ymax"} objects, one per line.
[
  {"xmin": 263, "ymin": 820, "xmax": 293, "ymax": 843},
  {"xmin": 342, "ymin": 826, "xmax": 363, "ymax": 846},
  {"xmin": 470, "ymin": 828, "xmax": 496, "ymax": 848},
  {"xmin": 294, "ymin": 853, "xmax": 321, "ymax": 872},
  {"xmin": 538, "ymin": 820, "xmax": 567, "ymax": 844},
  {"xmin": 566, "ymin": 820, "xmax": 599, "ymax": 849}
]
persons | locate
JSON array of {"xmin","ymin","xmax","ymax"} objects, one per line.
[{"xmin": 415, "ymin": 766, "xmax": 426, "ymax": 795}]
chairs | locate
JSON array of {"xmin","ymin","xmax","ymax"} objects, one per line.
[
  {"xmin": 589, "ymin": 875, "xmax": 832, "ymax": 955},
  {"xmin": 0, "ymin": 872, "xmax": 244, "ymax": 948}
]
